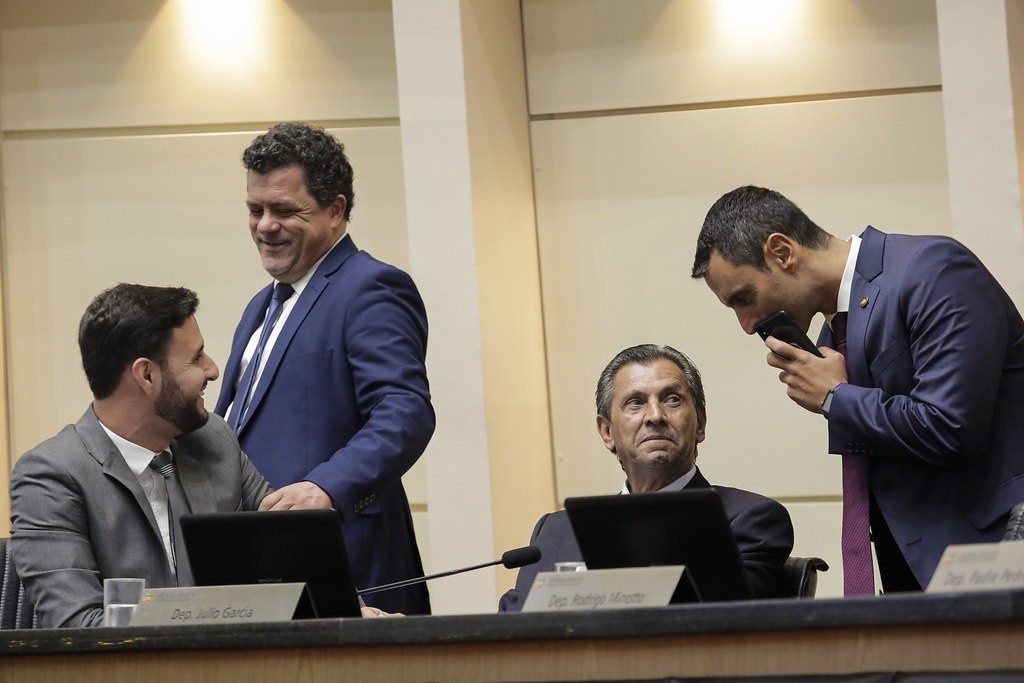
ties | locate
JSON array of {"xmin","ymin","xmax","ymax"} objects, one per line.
[
  {"xmin": 832, "ymin": 314, "xmax": 874, "ymax": 597},
  {"xmin": 151, "ymin": 452, "xmax": 197, "ymax": 587},
  {"xmin": 226, "ymin": 284, "xmax": 295, "ymax": 431}
]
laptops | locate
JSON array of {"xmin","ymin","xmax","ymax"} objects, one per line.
[
  {"xmin": 179, "ymin": 509, "xmax": 362, "ymax": 619},
  {"xmin": 565, "ymin": 489, "xmax": 758, "ymax": 597}
]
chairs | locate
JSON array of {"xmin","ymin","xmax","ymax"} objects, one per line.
[
  {"xmin": 771, "ymin": 557, "xmax": 831, "ymax": 598},
  {"xmin": 0, "ymin": 538, "xmax": 41, "ymax": 630}
]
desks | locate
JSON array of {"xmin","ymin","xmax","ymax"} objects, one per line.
[{"xmin": 1, "ymin": 591, "xmax": 1023, "ymax": 681}]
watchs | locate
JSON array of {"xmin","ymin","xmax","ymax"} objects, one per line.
[{"xmin": 819, "ymin": 381, "xmax": 847, "ymax": 418}]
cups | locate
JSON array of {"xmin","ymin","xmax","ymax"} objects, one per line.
[
  {"xmin": 554, "ymin": 561, "xmax": 588, "ymax": 571},
  {"xmin": 103, "ymin": 578, "xmax": 145, "ymax": 627}
]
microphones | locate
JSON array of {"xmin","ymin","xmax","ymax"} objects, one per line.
[{"xmin": 356, "ymin": 546, "xmax": 543, "ymax": 597}]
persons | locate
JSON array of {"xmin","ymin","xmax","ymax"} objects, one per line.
[
  {"xmin": 9, "ymin": 282, "xmax": 406, "ymax": 629},
  {"xmin": 211, "ymin": 120, "xmax": 436, "ymax": 615},
  {"xmin": 498, "ymin": 344, "xmax": 794, "ymax": 617},
  {"xmin": 692, "ymin": 185, "xmax": 1024, "ymax": 597}
]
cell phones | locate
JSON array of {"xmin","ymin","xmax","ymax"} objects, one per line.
[{"xmin": 756, "ymin": 308, "xmax": 824, "ymax": 361}]
